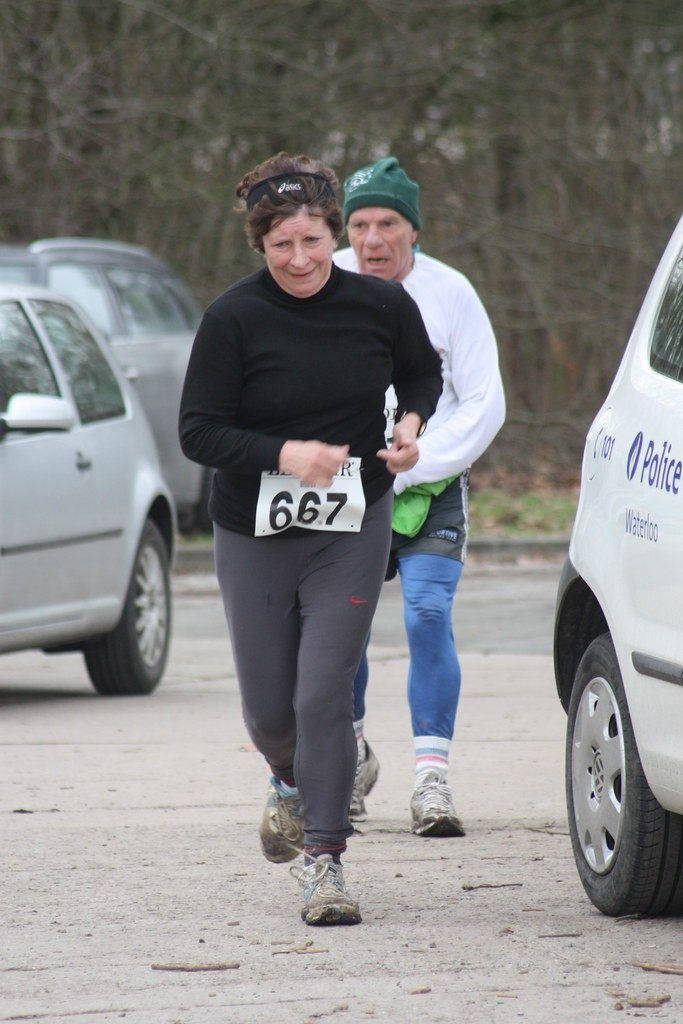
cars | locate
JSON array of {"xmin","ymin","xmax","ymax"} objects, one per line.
[
  {"xmin": 553, "ymin": 222, "xmax": 683, "ymax": 918},
  {"xmin": 0, "ymin": 285, "xmax": 178, "ymax": 695}
]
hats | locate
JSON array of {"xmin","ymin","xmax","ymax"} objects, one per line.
[{"xmin": 344, "ymin": 157, "xmax": 421, "ymax": 230}]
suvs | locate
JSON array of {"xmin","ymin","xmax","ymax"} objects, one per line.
[{"xmin": 0, "ymin": 236, "xmax": 225, "ymax": 543}]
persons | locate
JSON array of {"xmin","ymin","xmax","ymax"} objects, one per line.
[
  {"xmin": 333, "ymin": 157, "xmax": 506, "ymax": 837},
  {"xmin": 178, "ymin": 151, "xmax": 444, "ymax": 926}
]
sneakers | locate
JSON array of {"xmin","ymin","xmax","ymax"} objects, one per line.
[
  {"xmin": 287, "ymin": 843, "xmax": 362, "ymax": 926},
  {"xmin": 348, "ymin": 738, "xmax": 380, "ymax": 823},
  {"xmin": 260, "ymin": 776, "xmax": 307, "ymax": 862},
  {"xmin": 410, "ymin": 772, "xmax": 465, "ymax": 837}
]
